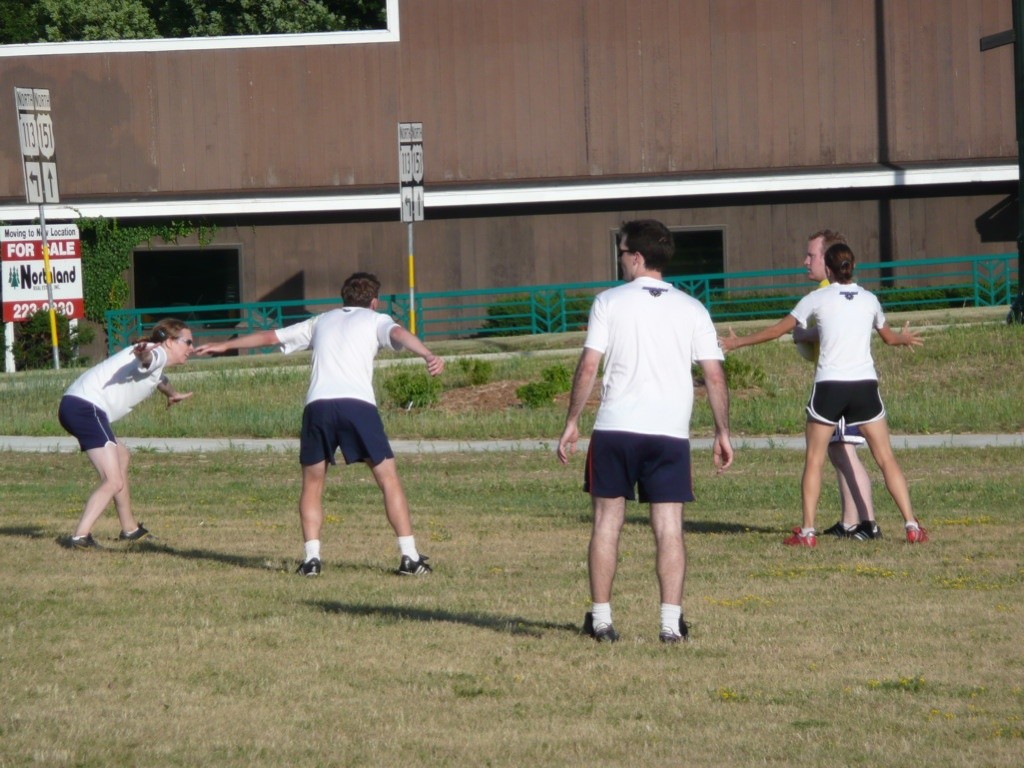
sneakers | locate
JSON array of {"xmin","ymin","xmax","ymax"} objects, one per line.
[
  {"xmin": 119, "ymin": 522, "xmax": 156, "ymax": 541},
  {"xmin": 818, "ymin": 521, "xmax": 859, "ymax": 537},
  {"xmin": 581, "ymin": 612, "xmax": 615, "ymax": 643},
  {"xmin": 784, "ymin": 526, "xmax": 815, "ymax": 547},
  {"xmin": 296, "ymin": 558, "xmax": 319, "ymax": 576},
  {"xmin": 658, "ymin": 614, "xmax": 692, "ymax": 643},
  {"xmin": 907, "ymin": 518, "xmax": 929, "ymax": 543},
  {"xmin": 849, "ymin": 525, "xmax": 883, "ymax": 542},
  {"xmin": 70, "ymin": 533, "xmax": 100, "ymax": 552},
  {"xmin": 400, "ymin": 555, "xmax": 432, "ymax": 576}
]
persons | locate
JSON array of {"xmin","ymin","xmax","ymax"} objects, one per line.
[
  {"xmin": 558, "ymin": 218, "xmax": 733, "ymax": 645},
  {"xmin": 59, "ymin": 317, "xmax": 194, "ymax": 555},
  {"xmin": 719, "ymin": 228, "xmax": 932, "ymax": 548},
  {"xmin": 191, "ymin": 272, "xmax": 445, "ymax": 579}
]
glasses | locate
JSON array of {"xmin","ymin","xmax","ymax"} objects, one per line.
[
  {"xmin": 619, "ymin": 250, "xmax": 632, "ymax": 257},
  {"xmin": 177, "ymin": 337, "xmax": 192, "ymax": 345}
]
